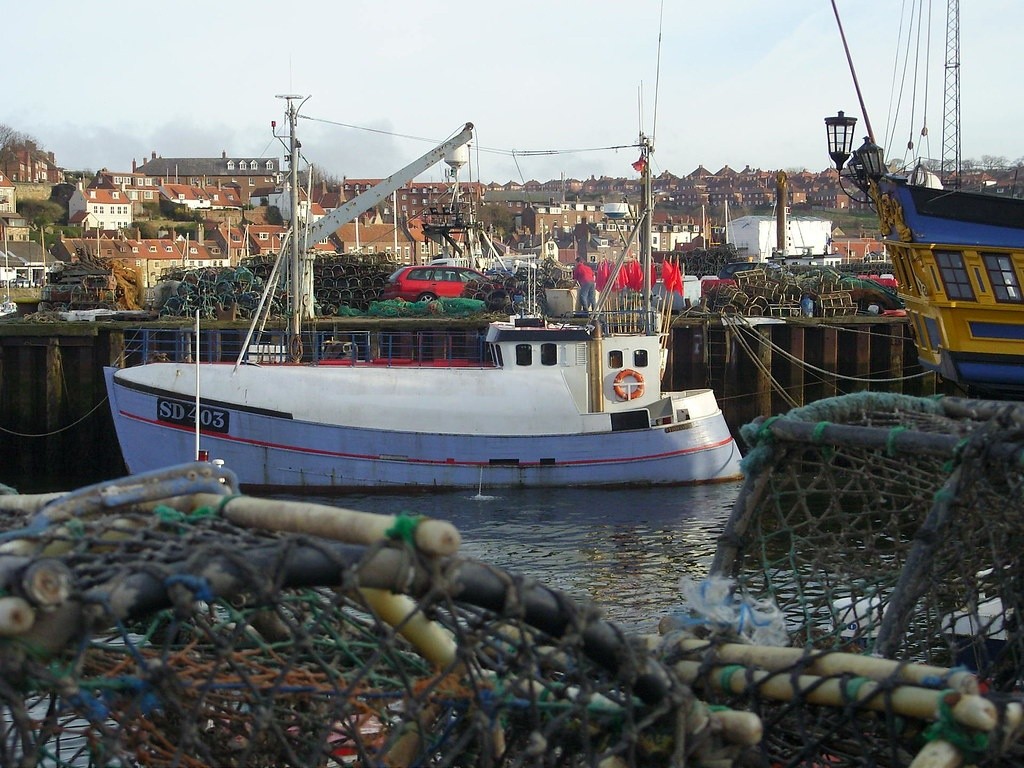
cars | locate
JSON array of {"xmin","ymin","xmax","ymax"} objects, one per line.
[{"xmin": 10, "ymin": 279, "xmax": 42, "ymax": 288}]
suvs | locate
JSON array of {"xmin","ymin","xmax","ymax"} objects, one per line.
[{"xmin": 379, "ymin": 264, "xmax": 500, "ymax": 304}]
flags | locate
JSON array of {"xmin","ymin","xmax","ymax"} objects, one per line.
[{"xmin": 595, "ymin": 257, "xmax": 684, "ymax": 297}]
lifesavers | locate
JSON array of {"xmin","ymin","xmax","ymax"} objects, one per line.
[{"xmin": 613, "ymin": 369, "xmax": 645, "ymax": 400}]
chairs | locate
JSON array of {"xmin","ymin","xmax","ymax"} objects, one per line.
[{"xmin": 444, "ymin": 273, "xmax": 463, "ymax": 282}]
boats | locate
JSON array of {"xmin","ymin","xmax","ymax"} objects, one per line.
[
  {"xmin": 867, "ymin": 0, "xmax": 1024, "ymax": 399},
  {"xmin": 101, "ymin": 124, "xmax": 746, "ymax": 496}
]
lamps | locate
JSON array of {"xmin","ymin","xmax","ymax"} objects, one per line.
[{"xmin": 825, "ymin": 110, "xmax": 891, "ymax": 236}]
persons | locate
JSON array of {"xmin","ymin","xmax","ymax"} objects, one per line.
[{"xmin": 569, "ymin": 256, "xmax": 596, "ymax": 318}]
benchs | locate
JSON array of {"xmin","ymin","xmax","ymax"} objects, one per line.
[{"xmin": 412, "ymin": 274, "xmax": 427, "ymax": 280}]
